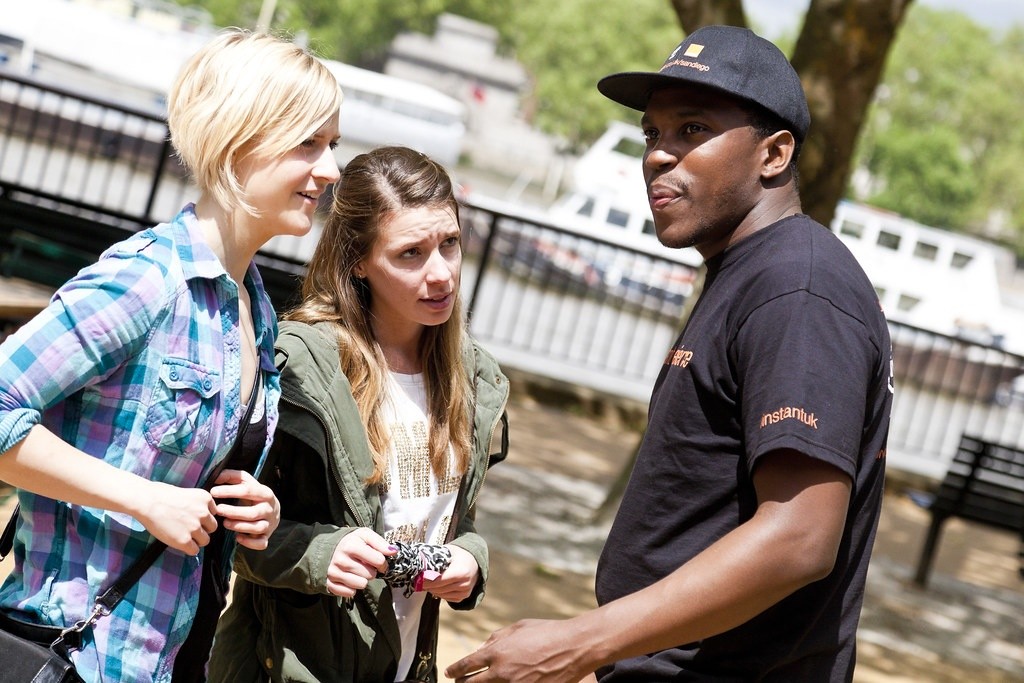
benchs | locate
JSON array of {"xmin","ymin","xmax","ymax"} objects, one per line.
[{"xmin": 909, "ymin": 431, "xmax": 1024, "ymax": 591}]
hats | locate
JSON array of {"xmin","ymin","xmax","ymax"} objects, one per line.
[{"xmin": 598, "ymin": 25, "xmax": 811, "ymax": 138}]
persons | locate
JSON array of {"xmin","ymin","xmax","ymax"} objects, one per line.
[
  {"xmin": 1, "ymin": 26, "xmax": 347, "ymax": 683},
  {"xmin": 206, "ymin": 148, "xmax": 511, "ymax": 683},
  {"xmin": 445, "ymin": 24, "xmax": 896, "ymax": 683}
]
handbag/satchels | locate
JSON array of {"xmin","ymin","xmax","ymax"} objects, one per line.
[{"xmin": 0, "ymin": 613, "xmax": 87, "ymax": 683}]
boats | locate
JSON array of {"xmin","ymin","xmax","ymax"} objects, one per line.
[
  {"xmin": 469, "ymin": 122, "xmax": 1024, "ymax": 405},
  {"xmin": 0, "ymin": 0, "xmax": 476, "ymax": 265}
]
